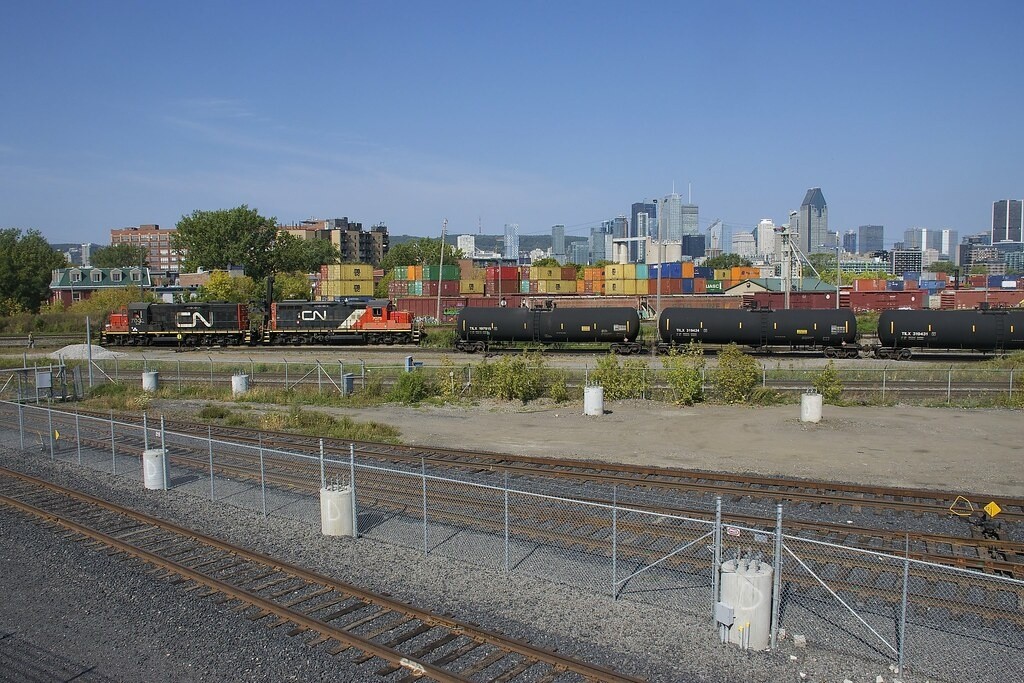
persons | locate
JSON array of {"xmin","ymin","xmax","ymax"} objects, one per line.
[
  {"xmin": 133, "ymin": 313, "xmax": 139, "ymax": 318},
  {"xmin": 26, "ymin": 331, "xmax": 34, "ymax": 348}
]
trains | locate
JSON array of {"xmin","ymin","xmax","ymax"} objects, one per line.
[{"xmin": 99, "ymin": 298, "xmax": 423, "ymax": 347}]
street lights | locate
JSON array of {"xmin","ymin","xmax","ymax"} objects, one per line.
[
  {"xmin": 653, "ymin": 198, "xmax": 668, "ymax": 340},
  {"xmin": 835, "ymin": 230, "xmax": 854, "ymax": 309},
  {"xmin": 437, "ymin": 218, "xmax": 448, "ymax": 326}
]
